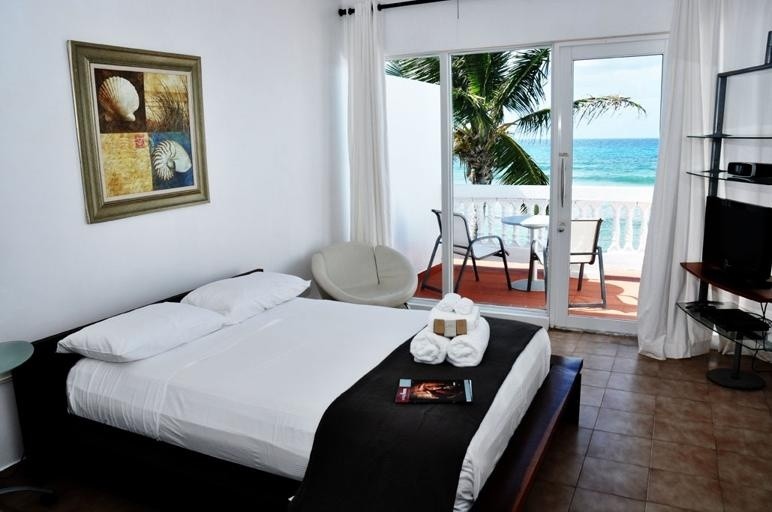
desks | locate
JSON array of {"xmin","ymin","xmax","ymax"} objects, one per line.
[
  {"xmin": 501, "ymin": 215, "xmax": 549, "ymax": 292},
  {"xmin": 0, "ymin": 341, "xmax": 56, "ymax": 505}
]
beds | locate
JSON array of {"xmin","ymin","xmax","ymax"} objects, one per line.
[{"xmin": 11, "ymin": 269, "xmax": 583, "ymax": 512}]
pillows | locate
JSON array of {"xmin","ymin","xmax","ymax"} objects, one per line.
[
  {"xmin": 55, "ymin": 302, "xmax": 226, "ymax": 363},
  {"xmin": 180, "ymin": 272, "xmax": 312, "ymax": 325}
]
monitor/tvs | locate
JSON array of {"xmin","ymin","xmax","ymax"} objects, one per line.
[{"xmin": 702, "ymin": 195, "xmax": 772, "ymax": 291}]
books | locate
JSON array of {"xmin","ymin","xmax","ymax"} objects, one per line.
[{"xmin": 395, "ymin": 377, "xmax": 474, "ymax": 404}]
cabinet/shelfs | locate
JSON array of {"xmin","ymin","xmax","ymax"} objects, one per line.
[{"xmin": 676, "ymin": 31, "xmax": 772, "ymax": 391}]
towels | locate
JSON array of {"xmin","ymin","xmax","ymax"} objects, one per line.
[{"xmin": 410, "ymin": 293, "xmax": 490, "ymax": 368}]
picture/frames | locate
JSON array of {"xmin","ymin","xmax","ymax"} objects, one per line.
[{"xmin": 67, "ymin": 39, "xmax": 210, "ymax": 224}]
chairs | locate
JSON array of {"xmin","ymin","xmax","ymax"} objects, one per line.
[
  {"xmin": 421, "ymin": 209, "xmax": 512, "ymax": 293},
  {"xmin": 311, "ymin": 241, "xmax": 419, "ymax": 309},
  {"xmin": 527, "ymin": 219, "xmax": 607, "ymax": 308}
]
